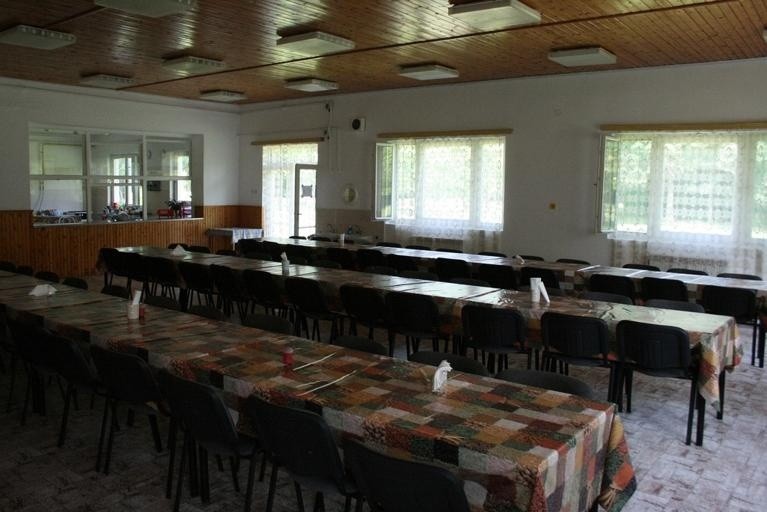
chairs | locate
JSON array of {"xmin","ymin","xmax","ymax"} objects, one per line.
[
  {"xmin": 105, "ymin": 204, "xmax": 141, "ymax": 222},
  {"xmin": 235, "ymin": 234, "xmax": 766, "ymax": 368},
  {"xmin": 45, "ymin": 214, "xmax": 79, "ymax": 224},
  {"xmin": 100, "ymin": 243, "xmax": 705, "ymax": 447},
  {"xmin": 2, "ymin": 261, "xmax": 594, "ymax": 512},
  {"xmin": 157, "ymin": 200, "xmax": 192, "ymax": 219}
]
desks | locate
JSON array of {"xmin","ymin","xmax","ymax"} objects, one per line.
[
  {"xmin": 0, "ymin": 227, "xmax": 767, "ymax": 512},
  {"xmin": 17, "ymin": 274, "xmax": 615, "ymax": 512},
  {"xmin": 106, "ymin": 247, "xmax": 731, "ymax": 445}
]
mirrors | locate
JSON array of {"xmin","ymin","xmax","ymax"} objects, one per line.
[{"xmin": 339, "ymin": 183, "xmax": 358, "ymax": 204}]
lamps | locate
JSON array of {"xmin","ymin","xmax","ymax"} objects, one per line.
[
  {"xmin": 400, "ymin": 65, "xmax": 459, "ymax": 81},
  {"xmin": 548, "ymin": 47, "xmax": 618, "ymax": 68},
  {"xmin": 94, "ymin": 0, "xmax": 196, "ymax": 18},
  {"xmin": 277, "ymin": 31, "xmax": 356, "ymax": 55},
  {"xmin": 0, "ymin": 25, "xmax": 77, "ymax": 50},
  {"xmin": 200, "ymin": 91, "xmax": 246, "ymax": 103},
  {"xmin": 448, "ymin": 0, "xmax": 542, "ymax": 33},
  {"xmin": 283, "ymin": 79, "xmax": 338, "ymax": 92},
  {"xmin": 80, "ymin": 74, "xmax": 136, "ymax": 90},
  {"xmin": 161, "ymin": 55, "xmax": 225, "ymax": 77}
]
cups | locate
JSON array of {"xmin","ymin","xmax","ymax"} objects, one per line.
[
  {"xmin": 282, "ymin": 346, "xmax": 293, "ymax": 364},
  {"xmin": 529, "ymin": 288, "xmax": 540, "ymax": 302},
  {"xmin": 431, "ymin": 371, "xmax": 446, "ymax": 395},
  {"xmin": 126, "ymin": 302, "xmax": 140, "ymax": 320},
  {"xmin": 139, "ymin": 302, "xmax": 144, "ymax": 316},
  {"xmin": 282, "ymin": 260, "xmax": 289, "ymax": 271},
  {"xmin": 338, "ymin": 234, "xmax": 344, "ymax": 241}
]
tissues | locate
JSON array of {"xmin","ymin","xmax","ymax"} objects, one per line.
[
  {"xmin": 530, "ymin": 278, "xmax": 550, "ymax": 303},
  {"xmin": 127, "ymin": 291, "xmax": 142, "ymax": 319},
  {"xmin": 281, "ymin": 253, "xmax": 290, "ymax": 267},
  {"xmin": 339, "ymin": 234, "xmax": 346, "ymax": 242},
  {"xmin": 30, "ymin": 284, "xmax": 56, "ymax": 295},
  {"xmin": 174, "ymin": 246, "xmax": 195, "ymax": 261},
  {"xmin": 516, "ymin": 255, "xmax": 525, "ymax": 265},
  {"xmin": 429, "ymin": 360, "xmax": 454, "ymax": 392}
]
